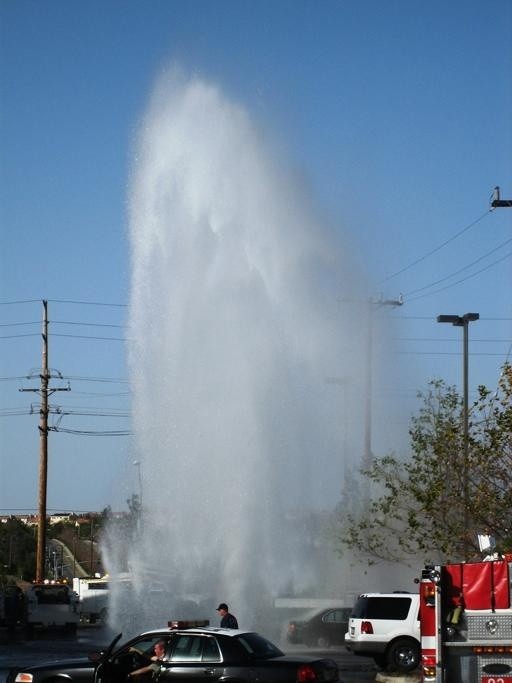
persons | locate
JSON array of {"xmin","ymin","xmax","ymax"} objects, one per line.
[
  {"xmin": 216, "ymin": 602, "xmax": 239, "ymax": 628},
  {"xmin": 126, "ymin": 639, "xmax": 169, "ymax": 682}
]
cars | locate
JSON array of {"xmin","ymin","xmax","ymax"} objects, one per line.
[
  {"xmin": 286, "ymin": 607, "xmax": 352, "ymax": 649},
  {"xmin": 5, "ymin": 617, "xmax": 337, "ymax": 683},
  {"xmin": 20, "ymin": 583, "xmax": 80, "ymax": 641},
  {"xmin": 344, "ymin": 593, "xmax": 421, "ymax": 672}
]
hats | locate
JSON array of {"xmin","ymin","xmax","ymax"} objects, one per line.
[{"xmin": 216, "ymin": 603, "xmax": 227, "ymax": 610}]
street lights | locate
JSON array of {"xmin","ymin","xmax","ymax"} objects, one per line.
[{"xmin": 436, "ymin": 312, "xmax": 479, "ymax": 562}]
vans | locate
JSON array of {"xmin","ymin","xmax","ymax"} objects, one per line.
[{"xmin": 71, "ymin": 577, "xmax": 134, "ymax": 624}]
